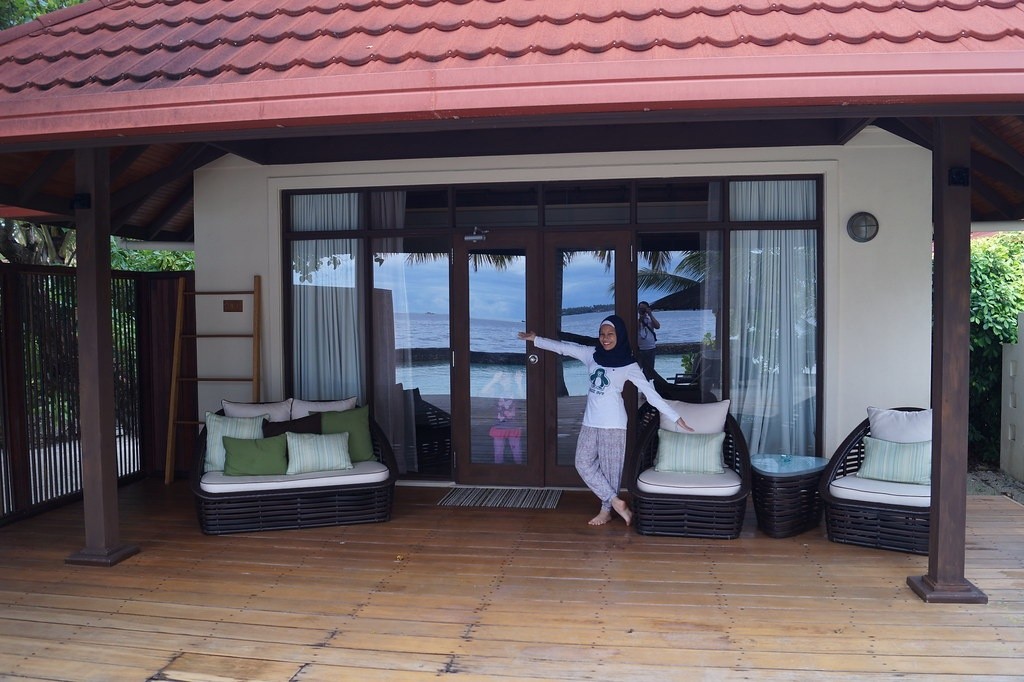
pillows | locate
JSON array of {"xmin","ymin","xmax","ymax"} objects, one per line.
[
  {"xmin": 203, "ymin": 411, "xmax": 270, "ymax": 471},
  {"xmin": 654, "ymin": 429, "xmax": 726, "ymax": 475},
  {"xmin": 222, "ymin": 435, "xmax": 287, "ymax": 476},
  {"xmin": 292, "ymin": 396, "xmax": 356, "ymax": 421},
  {"xmin": 867, "ymin": 406, "xmax": 932, "ymax": 444},
  {"xmin": 405, "ymin": 387, "xmax": 430, "ymax": 427},
  {"xmin": 262, "ymin": 413, "xmax": 321, "ymax": 437},
  {"xmin": 857, "ymin": 436, "xmax": 932, "ymax": 485},
  {"xmin": 285, "ymin": 432, "xmax": 353, "ymax": 474},
  {"xmin": 308, "ymin": 403, "xmax": 377, "ymax": 462},
  {"xmin": 221, "ymin": 398, "xmax": 293, "ymax": 421},
  {"xmin": 658, "ymin": 398, "xmax": 730, "ymax": 467}
]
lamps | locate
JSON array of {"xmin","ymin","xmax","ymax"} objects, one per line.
[
  {"xmin": 465, "ymin": 226, "xmax": 491, "ymax": 243},
  {"xmin": 847, "ymin": 210, "xmax": 879, "ymax": 243}
]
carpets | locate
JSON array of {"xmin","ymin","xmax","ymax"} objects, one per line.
[{"xmin": 435, "ymin": 487, "xmax": 563, "ymax": 512}]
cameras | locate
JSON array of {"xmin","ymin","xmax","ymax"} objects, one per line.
[{"xmin": 638, "ymin": 308, "xmax": 647, "ymax": 315}]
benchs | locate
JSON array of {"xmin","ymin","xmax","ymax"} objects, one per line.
[
  {"xmin": 191, "ymin": 399, "xmax": 399, "ymax": 534},
  {"xmin": 396, "ymin": 383, "xmax": 452, "ymax": 471}
]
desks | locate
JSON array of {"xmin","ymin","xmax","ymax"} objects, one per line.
[{"xmin": 751, "ymin": 454, "xmax": 830, "ymax": 537}]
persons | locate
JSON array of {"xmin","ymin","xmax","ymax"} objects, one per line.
[
  {"xmin": 518, "ymin": 315, "xmax": 694, "ymax": 528},
  {"xmin": 636, "ymin": 302, "xmax": 661, "ymax": 368},
  {"xmin": 484, "ymin": 365, "xmax": 526, "ymax": 464}
]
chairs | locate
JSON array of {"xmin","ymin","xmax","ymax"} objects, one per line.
[
  {"xmin": 625, "ymin": 390, "xmax": 751, "ymax": 539},
  {"xmin": 817, "ymin": 407, "xmax": 933, "ymax": 554}
]
